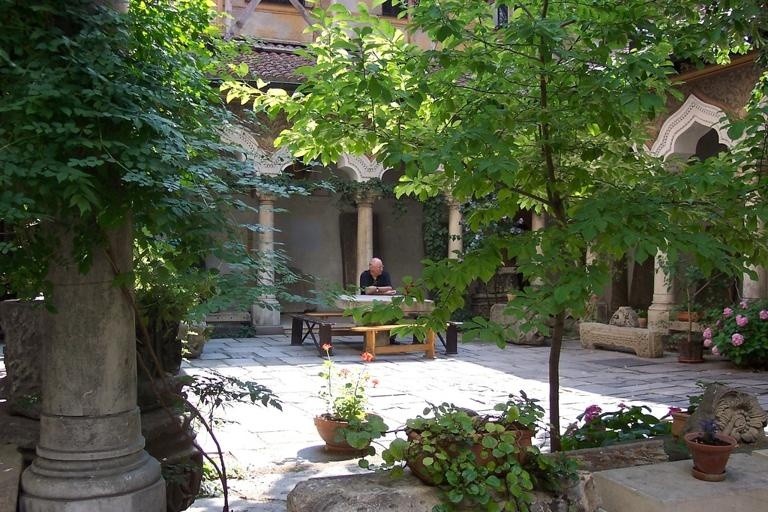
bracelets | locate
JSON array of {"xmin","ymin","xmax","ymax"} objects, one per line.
[{"xmin": 376, "ymin": 287, "xmax": 379, "ymax": 293}]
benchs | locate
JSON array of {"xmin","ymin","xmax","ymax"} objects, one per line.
[{"xmin": 287, "ymin": 308, "xmax": 464, "ymax": 361}]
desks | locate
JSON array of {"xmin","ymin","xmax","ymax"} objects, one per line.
[{"xmin": 336, "ymin": 290, "xmax": 436, "ymax": 347}]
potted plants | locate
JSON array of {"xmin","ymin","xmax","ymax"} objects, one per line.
[
  {"xmin": 658, "ymin": 244, "xmax": 725, "ymax": 364},
  {"xmin": 670, "ymin": 379, "xmax": 733, "ymax": 440},
  {"xmin": 363, "ymin": 389, "xmax": 578, "ymax": 512}
]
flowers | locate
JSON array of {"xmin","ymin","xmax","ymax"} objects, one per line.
[
  {"xmin": 546, "ymin": 401, "xmax": 679, "ymax": 449},
  {"xmin": 313, "ymin": 340, "xmax": 379, "ymax": 418},
  {"xmin": 694, "ymin": 411, "xmax": 722, "ymax": 443},
  {"xmin": 702, "ymin": 297, "xmax": 768, "ymax": 369}
]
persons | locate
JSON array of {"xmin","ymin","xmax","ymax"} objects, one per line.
[{"xmin": 359, "ymin": 258, "xmax": 401, "ymax": 345}]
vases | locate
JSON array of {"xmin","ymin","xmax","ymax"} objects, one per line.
[
  {"xmin": 315, "ymin": 410, "xmax": 384, "ymax": 454},
  {"xmin": 683, "ymin": 432, "xmax": 736, "ymax": 475}
]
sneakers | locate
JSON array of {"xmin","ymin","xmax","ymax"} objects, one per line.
[{"xmin": 390, "ymin": 337, "xmax": 400, "ymax": 345}]
